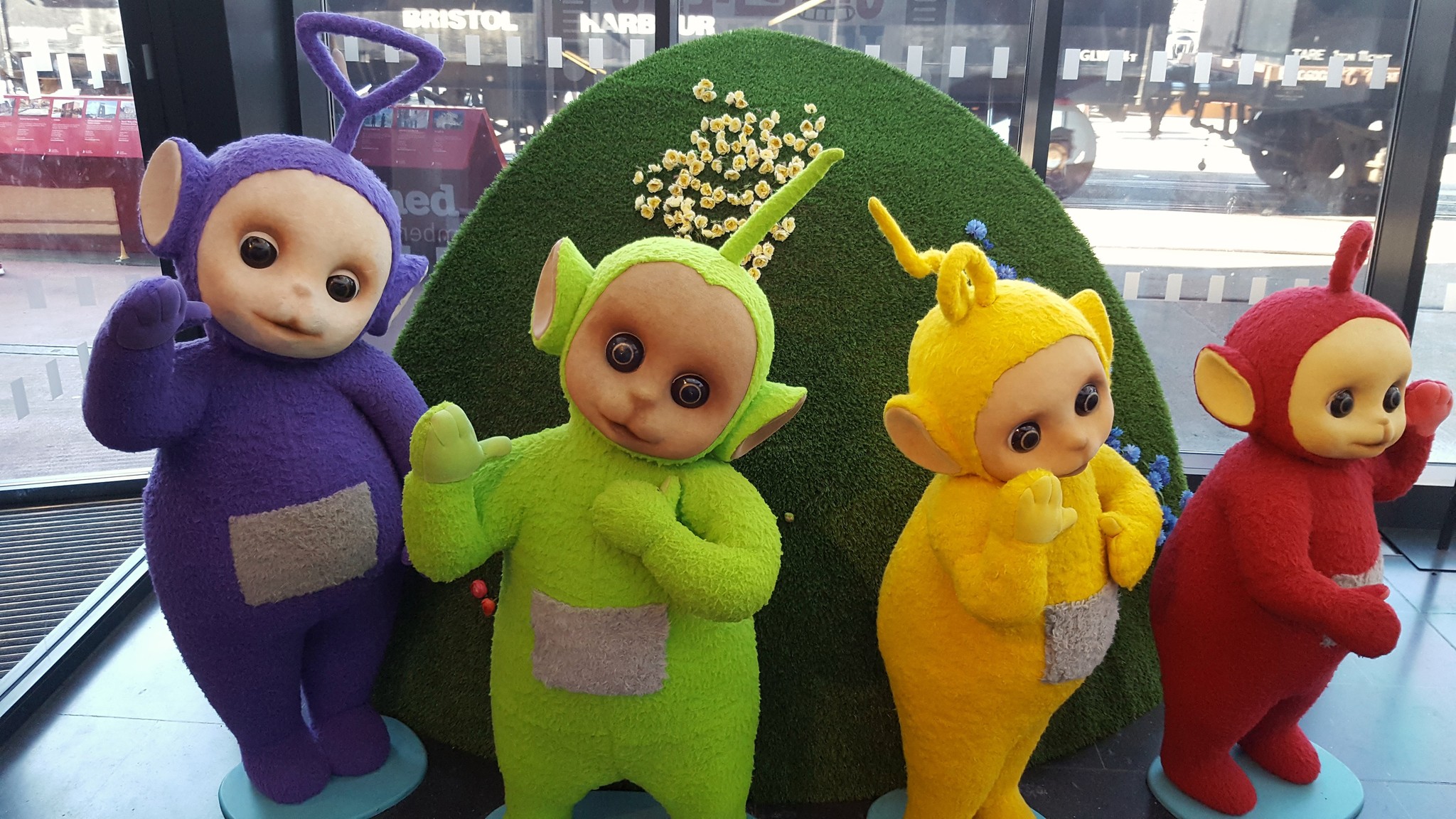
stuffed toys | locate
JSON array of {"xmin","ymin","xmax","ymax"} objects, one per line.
[
  {"xmin": 867, "ymin": 194, "xmax": 1163, "ymax": 819},
  {"xmin": 402, "ymin": 145, "xmax": 845, "ymax": 819},
  {"xmin": 1148, "ymin": 221, "xmax": 1453, "ymax": 815},
  {"xmin": 82, "ymin": 12, "xmax": 445, "ymax": 804}
]
